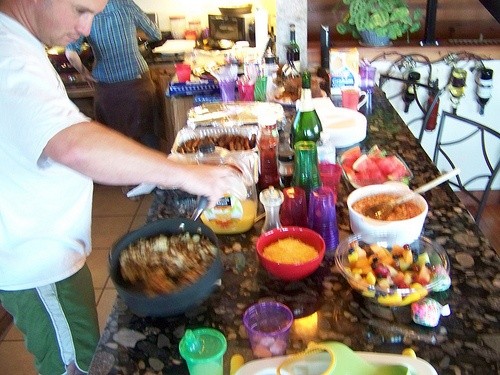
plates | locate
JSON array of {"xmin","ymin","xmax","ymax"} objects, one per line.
[
  {"xmin": 187, "ymin": 100, "xmax": 284, "ymax": 126},
  {"xmin": 320, "ymin": 107, "xmax": 367, "ymax": 149},
  {"xmin": 337, "ymin": 149, "xmax": 414, "ymax": 191}
]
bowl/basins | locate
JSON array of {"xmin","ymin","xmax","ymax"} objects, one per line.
[
  {"xmin": 256, "ymin": 226, "xmax": 325, "ymax": 282},
  {"xmin": 346, "ymin": 184, "xmax": 429, "ymax": 248},
  {"xmin": 334, "ymin": 228, "xmax": 451, "ymax": 308},
  {"xmin": 171, "ymin": 126, "xmax": 258, "ymax": 155}
]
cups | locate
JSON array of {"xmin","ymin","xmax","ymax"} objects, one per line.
[
  {"xmin": 170, "ymin": 16, "xmax": 202, "ymax": 39},
  {"xmin": 243, "ymin": 302, "xmax": 293, "ymax": 360},
  {"xmin": 280, "ymin": 164, "xmax": 341, "ymax": 251},
  {"xmin": 340, "ymin": 65, "xmax": 376, "ymax": 112},
  {"xmin": 175, "ymin": 64, "xmax": 191, "ymax": 83},
  {"xmin": 218, "ymin": 77, "xmax": 267, "ymax": 103},
  {"xmin": 178, "ymin": 328, "xmax": 227, "ymax": 375}
]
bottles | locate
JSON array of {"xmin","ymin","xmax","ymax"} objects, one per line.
[
  {"xmin": 262, "ymin": 23, "xmax": 301, "ymax": 74},
  {"xmin": 290, "ymin": 71, "xmax": 325, "ymax": 151},
  {"xmin": 257, "ymin": 119, "xmax": 321, "ymax": 213},
  {"xmin": 259, "ymin": 185, "xmax": 284, "ymax": 236},
  {"xmin": 410, "ymin": 274, "xmax": 450, "ymax": 335},
  {"xmin": 402, "ymin": 67, "xmax": 494, "ymax": 131},
  {"xmin": 196, "ymin": 143, "xmax": 257, "ymax": 234}
]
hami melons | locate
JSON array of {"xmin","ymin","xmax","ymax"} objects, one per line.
[{"xmin": 346, "ymin": 243, "xmax": 431, "ymax": 305}]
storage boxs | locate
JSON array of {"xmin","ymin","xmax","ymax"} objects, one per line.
[{"xmin": 329, "ymin": 49, "xmax": 361, "ymax": 95}]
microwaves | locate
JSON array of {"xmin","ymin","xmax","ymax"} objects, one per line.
[{"xmin": 207, "ymin": 13, "xmax": 254, "ymax": 42}]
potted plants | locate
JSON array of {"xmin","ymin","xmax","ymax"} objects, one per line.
[{"xmin": 336, "ymin": 0, "xmax": 424, "ymax": 46}]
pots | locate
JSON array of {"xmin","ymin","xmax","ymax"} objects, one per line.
[{"xmin": 106, "ymin": 196, "xmax": 223, "ymax": 320}]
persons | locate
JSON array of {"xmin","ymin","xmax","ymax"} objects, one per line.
[
  {"xmin": 65, "ymin": 0, "xmax": 162, "ymax": 200},
  {"xmin": 0, "ymin": 0, "xmax": 249, "ymax": 375}
]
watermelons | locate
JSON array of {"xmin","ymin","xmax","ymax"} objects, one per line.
[{"xmin": 343, "ymin": 144, "xmax": 407, "ymax": 187}]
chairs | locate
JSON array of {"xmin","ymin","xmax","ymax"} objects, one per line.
[
  {"xmin": 379, "ymin": 75, "xmax": 441, "ymax": 142},
  {"xmin": 431, "ymin": 110, "xmax": 500, "ymax": 225}
]
